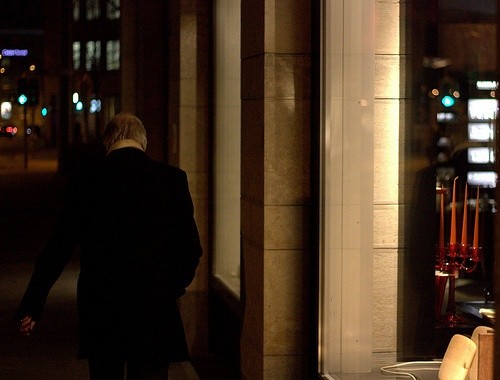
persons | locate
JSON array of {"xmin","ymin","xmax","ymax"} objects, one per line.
[{"xmin": 10, "ymin": 113, "xmax": 204, "ymax": 380}]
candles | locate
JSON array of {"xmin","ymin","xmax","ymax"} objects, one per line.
[{"xmin": 437, "ymin": 178, "xmax": 481, "ymax": 252}]
